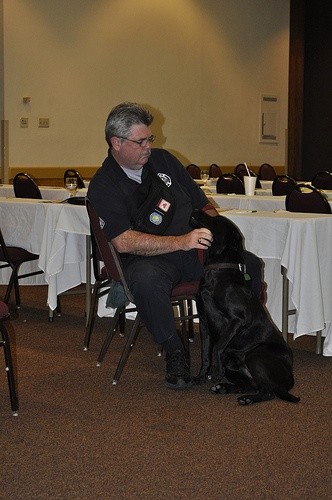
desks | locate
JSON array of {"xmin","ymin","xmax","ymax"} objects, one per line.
[{"xmin": 0, "ymin": 180, "xmax": 332, "ymax": 356}]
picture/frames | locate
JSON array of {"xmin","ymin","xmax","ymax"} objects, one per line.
[{"xmin": 258, "ymin": 93, "xmax": 280, "ymax": 143}]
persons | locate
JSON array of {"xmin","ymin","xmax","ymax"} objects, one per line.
[{"xmin": 85, "ymin": 102, "xmax": 264, "ymax": 389}]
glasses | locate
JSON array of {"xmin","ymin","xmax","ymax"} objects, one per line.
[{"xmin": 110, "ymin": 135, "xmax": 155, "ymax": 147}]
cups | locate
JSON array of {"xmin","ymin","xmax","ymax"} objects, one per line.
[{"xmin": 244, "ymin": 176, "xmax": 257, "ymax": 196}]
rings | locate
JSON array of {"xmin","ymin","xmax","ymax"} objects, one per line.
[{"xmin": 199, "ymin": 238, "xmax": 201, "ymax": 242}]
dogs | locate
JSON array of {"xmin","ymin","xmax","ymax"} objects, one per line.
[{"xmin": 190, "ymin": 208, "xmax": 301, "ymax": 405}]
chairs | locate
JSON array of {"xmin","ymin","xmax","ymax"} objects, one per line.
[
  {"xmin": 13, "ymin": 173, "xmax": 42, "ymax": 199},
  {"xmin": 64, "ymin": 161, "xmax": 331, "ymax": 385},
  {"xmin": 1, "ymin": 232, "xmax": 43, "ymax": 411}
]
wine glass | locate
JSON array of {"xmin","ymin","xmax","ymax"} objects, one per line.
[
  {"xmin": 65, "ymin": 177, "xmax": 77, "ymax": 198},
  {"xmin": 200, "ymin": 170, "xmax": 209, "ymax": 188}
]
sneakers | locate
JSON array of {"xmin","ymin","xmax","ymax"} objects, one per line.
[{"xmin": 165, "ymin": 344, "xmax": 192, "ymax": 389}]
